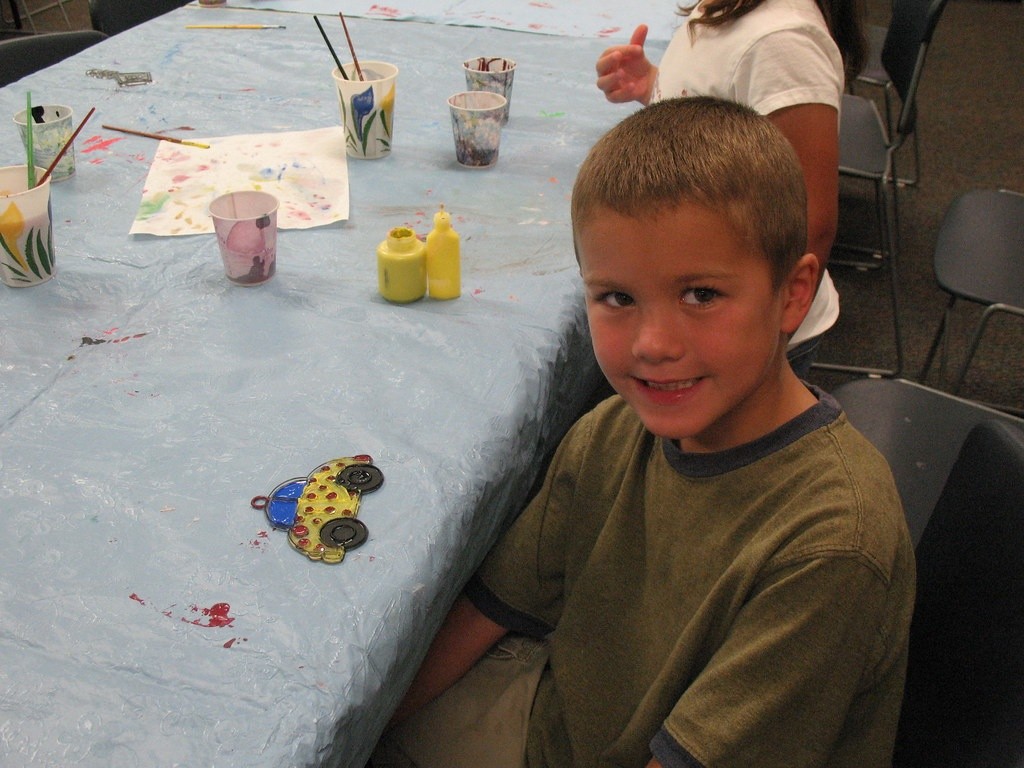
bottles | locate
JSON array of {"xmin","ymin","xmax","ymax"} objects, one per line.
[
  {"xmin": 425, "ymin": 212, "xmax": 462, "ymax": 301},
  {"xmin": 376, "ymin": 226, "xmax": 428, "ymax": 305}
]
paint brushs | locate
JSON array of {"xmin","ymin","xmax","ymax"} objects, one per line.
[
  {"xmin": 185, "ymin": 24, "xmax": 286, "ymax": 29},
  {"xmin": 313, "ymin": 15, "xmax": 348, "ymax": 80},
  {"xmin": 26, "ymin": 91, "xmax": 35, "ymax": 189},
  {"xmin": 339, "ymin": 12, "xmax": 364, "ymax": 81},
  {"xmin": 37, "ymin": 107, "xmax": 96, "ymax": 186},
  {"xmin": 101, "ymin": 125, "xmax": 210, "ymax": 149}
]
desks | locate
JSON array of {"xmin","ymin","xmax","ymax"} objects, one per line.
[{"xmin": 0, "ymin": 0, "xmax": 701, "ymax": 768}]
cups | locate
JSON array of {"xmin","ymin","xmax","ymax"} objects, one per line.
[
  {"xmin": 331, "ymin": 61, "xmax": 400, "ymax": 160},
  {"xmin": 447, "ymin": 91, "xmax": 509, "ymax": 169},
  {"xmin": 0, "ymin": 164, "xmax": 57, "ymax": 288},
  {"xmin": 463, "ymin": 57, "xmax": 516, "ymax": 128},
  {"xmin": 209, "ymin": 190, "xmax": 280, "ymax": 287},
  {"xmin": 13, "ymin": 104, "xmax": 76, "ymax": 184}
]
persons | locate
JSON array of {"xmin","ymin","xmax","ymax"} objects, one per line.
[
  {"xmin": 595, "ymin": 0, "xmax": 872, "ymax": 379},
  {"xmin": 364, "ymin": 96, "xmax": 916, "ymax": 768}
]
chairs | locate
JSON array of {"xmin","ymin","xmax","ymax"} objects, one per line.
[{"xmin": 807, "ymin": 0, "xmax": 1024, "ymax": 768}]
